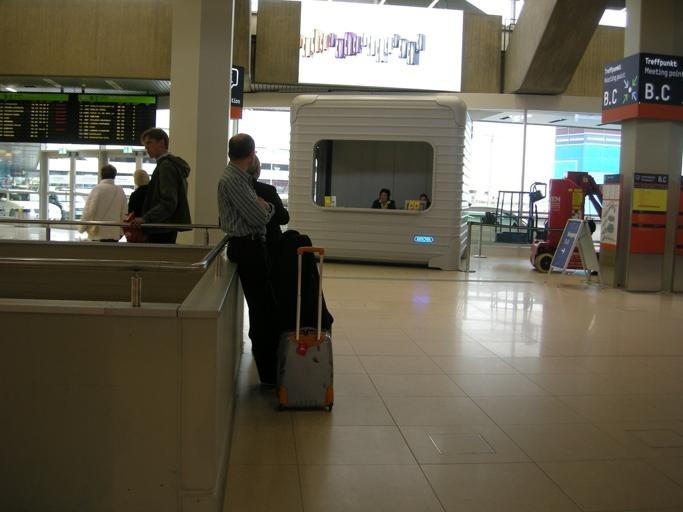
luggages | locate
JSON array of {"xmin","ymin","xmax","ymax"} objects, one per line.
[{"xmin": 275, "ymin": 246, "xmax": 334, "ymax": 413}]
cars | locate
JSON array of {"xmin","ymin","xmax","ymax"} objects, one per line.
[
  {"xmin": 461, "ymin": 206, "xmax": 527, "ymax": 242},
  {"xmin": 0, "ymin": 185, "xmax": 85, "ymax": 227}
]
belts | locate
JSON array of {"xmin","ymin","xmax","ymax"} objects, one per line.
[{"xmin": 248, "ymin": 233, "xmax": 266, "ymax": 240}]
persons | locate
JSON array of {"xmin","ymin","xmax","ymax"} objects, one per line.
[
  {"xmin": 126, "ymin": 168, "xmax": 151, "ymax": 218},
  {"xmin": 245, "ymin": 153, "xmax": 289, "ymax": 247},
  {"xmin": 77, "ymin": 163, "xmax": 129, "ymax": 241},
  {"xmin": 217, "ymin": 132, "xmax": 282, "ymax": 388},
  {"xmin": 370, "ymin": 188, "xmax": 395, "ymax": 209},
  {"xmin": 131, "ymin": 128, "xmax": 193, "ymax": 245},
  {"xmin": 418, "ymin": 194, "xmax": 431, "ymax": 211}
]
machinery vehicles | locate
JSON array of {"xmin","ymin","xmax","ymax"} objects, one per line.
[{"xmin": 528, "ymin": 170, "xmax": 603, "ymax": 273}]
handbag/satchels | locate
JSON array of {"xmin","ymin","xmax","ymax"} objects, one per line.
[{"xmin": 86, "ymin": 225, "xmax": 98, "ymax": 235}]
